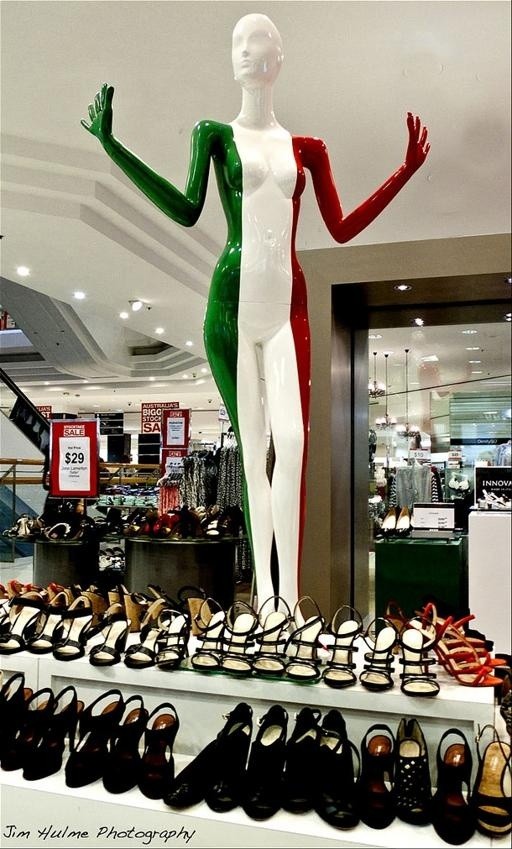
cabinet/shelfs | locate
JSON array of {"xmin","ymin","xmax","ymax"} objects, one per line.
[
  {"xmin": 468, "ymin": 511, "xmax": 512, "ymax": 659},
  {"xmin": 375, "ymin": 537, "xmax": 468, "ymax": 635},
  {"xmin": 0, "ymin": 602, "xmax": 511, "ymax": 848}
]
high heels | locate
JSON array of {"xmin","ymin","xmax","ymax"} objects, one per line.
[
  {"xmin": 241, "ymin": 705, "xmax": 288, "ymax": 820},
  {"xmin": 206, "ymin": 504, "xmax": 248, "ymax": 539},
  {"xmin": 220, "ymin": 600, "xmax": 258, "ymax": 672},
  {"xmin": 358, "ymin": 724, "xmax": 395, "ymax": 828},
  {"xmin": 359, "ymin": 617, "xmax": 399, "ymax": 692},
  {"xmin": 0, "ymin": 582, "xmax": 8, "ymax": 599},
  {"xmin": 154, "ymin": 607, "xmax": 191, "ymax": 669},
  {"xmin": 28, "ymin": 591, "xmax": 70, "ymax": 654},
  {"xmin": 399, "ymin": 616, "xmax": 439, "ymax": 696},
  {"xmin": 88, "ymin": 603, "xmax": 132, "ymax": 666},
  {"xmin": 0, "ymin": 591, "xmax": 44, "ymax": 655},
  {"xmin": 65, "ymin": 689, "xmax": 126, "ymax": 787},
  {"xmin": 124, "ymin": 610, "xmax": 171, "ymax": 669},
  {"xmin": 81, "ymin": 585, "xmax": 109, "ymax": 626},
  {"xmin": 432, "ymin": 728, "xmax": 475, "ymax": 845},
  {"xmin": 380, "ymin": 507, "xmax": 396, "ymax": 535},
  {"xmin": 1, "ymin": 688, "xmax": 58, "ymax": 770},
  {"xmin": 383, "ymin": 601, "xmax": 411, "ymax": 655},
  {"xmin": 192, "ymin": 597, "xmax": 227, "ymax": 670},
  {"xmin": 121, "ymin": 584, "xmax": 145, "ymax": 632},
  {"xmin": 286, "ymin": 595, "xmax": 325, "ymax": 680},
  {"xmin": 396, "ymin": 505, "xmax": 410, "ymax": 535},
  {"xmin": 22, "ymin": 686, "xmax": 84, "ymax": 780},
  {"xmin": 282, "ymin": 707, "xmax": 323, "ymax": 813},
  {"xmin": 106, "ymin": 586, "xmax": 123, "ymax": 615},
  {"xmin": 252, "ymin": 595, "xmax": 291, "ymax": 673},
  {"xmin": 406, "ymin": 616, "xmax": 504, "ymax": 686},
  {"xmin": 3, "ymin": 513, "xmax": 83, "ymax": 543},
  {"xmin": 103, "ymin": 695, "xmax": 148, "ymax": 793},
  {"xmin": 138, "ymin": 703, "xmax": 179, "ymax": 799},
  {"xmin": 22, "ymin": 584, "xmax": 31, "ymax": 593},
  {"xmin": 47, "ymin": 583, "xmax": 59, "ymax": 600},
  {"xmin": 57, "ymin": 585, "xmax": 64, "ymax": 593},
  {"xmin": 53, "ymin": 596, "xmax": 95, "ymax": 660},
  {"xmin": 84, "ymin": 505, "xmax": 204, "ymax": 539},
  {"xmin": 31, "ymin": 586, "xmax": 48, "ymax": 601},
  {"xmin": 178, "ymin": 585, "xmax": 212, "ymax": 635},
  {"xmin": 165, "ymin": 739, "xmax": 216, "ymax": 808},
  {"xmin": 478, "ymin": 488, "xmax": 512, "ymax": 509},
  {"xmin": 0, "ymin": 673, "xmax": 32, "ymax": 724},
  {"xmin": 393, "ymin": 718, "xmax": 432, "ymax": 825},
  {"xmin": 131, "ymin": 585, "xmax": 177, "ymax": 629},
  {"xmin": 9, "ymin": 580, "xmax": 23, "ymax": 597},
  {"xmin": 314, "ymin": 709, "xmax": 360, "ymax": 829},
  {"xmin": 472, "ymin": 724, "xmax": 512, "ymax": 839},
  {"xmin": 416, "ymin": 603, "xmax": 507, "ymax": 666},
  {"xmin": 322, "ymin": 604, "xmax": 363, "ymax": 687},
  {"xmin": 204, "ymin": 703, "xmax": 252, "ymax": 812},
  {"xmin": 66, "ymin": 584, "xmax": 81, "ymax": 600}
]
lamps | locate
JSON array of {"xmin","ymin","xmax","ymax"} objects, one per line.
[
  {"xmin": 397, "ymin": 349, "xmax": 420, "ymax": 437},
  {"xmin": 130, "ymin": 301, "xmax": 142, "ymax": 312},
  {"xmin": 368, "ymin": 352, "xmax": 385, "ymax": 397},
  {"xmin": 375, "ymin": 354, "xmax": 397, "ymax": 430}
]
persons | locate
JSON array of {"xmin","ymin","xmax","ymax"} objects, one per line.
[
  {"xmin": 43, "ymin": 448, "xmax": 90, "ymax": 514},
  {"xmin": 78, "ymin": 13, "xmax": 432, "ymax": 631}
]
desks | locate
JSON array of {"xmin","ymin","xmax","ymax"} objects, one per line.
[
  {"xmin": 126, "ymin": 532, "xmax": 245, "ymax": 615},
  {"xmin": 14, "ymin": 534, "xmax": 118, "ymax": 590}
]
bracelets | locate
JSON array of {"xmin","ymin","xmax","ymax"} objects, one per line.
[{"xmin": 78, "ymin": 502, "xmax": 84, "ymax": 507}]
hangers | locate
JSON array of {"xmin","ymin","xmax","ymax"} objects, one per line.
[
  {"xmin": 156, "ymin": 462, "xmax": 184, "ymax": 487},
  {"xmin": 181, "ymin": 440, "xmax": 238, "ymax": 462}
]
